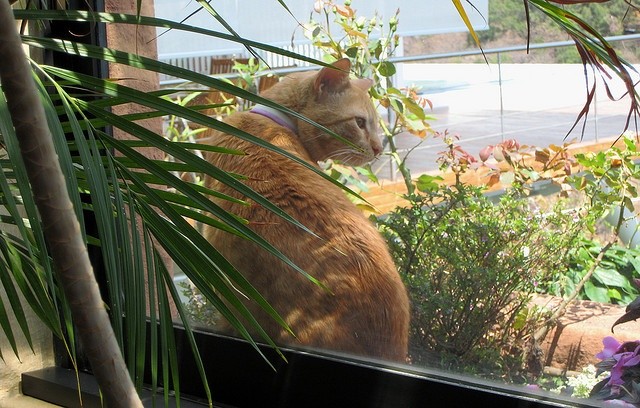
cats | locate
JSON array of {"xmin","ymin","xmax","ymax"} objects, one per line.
[{"xmin": 199, "ymin": 57, "xmax": 412, "ymax": 364}]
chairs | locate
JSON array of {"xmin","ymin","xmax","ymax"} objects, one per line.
[
  {"xmin": 210, "ymin": 56, "xmax": 231, "ymax": 73},
  {"xmin": 237, "ymin": 58, "xmax": 259, "ymax": 70},
  {"xmin": 207, "ymin": 77, "xmax": 249, "ymax": 123},
  {"xmin": 258, "ymin": 74, "xmax": 278, "ymax": 93}
]
